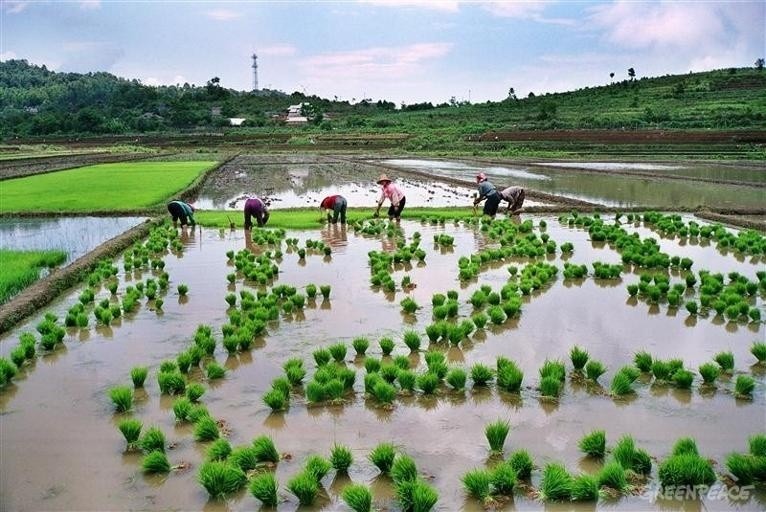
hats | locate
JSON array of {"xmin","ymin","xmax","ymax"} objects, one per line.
[{"xmin": 377, "ymin": 175, "xmax": 391, "ymax": 184}]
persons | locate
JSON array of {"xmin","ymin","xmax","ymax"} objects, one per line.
[
  {"xmin": 496, "ymin": 185, "xmax": 525, "ymax": 214},
  {"xmin": 322, "ymin": 222, "xmax": 349, "ymax": 248},
  {"xmin": 242, "ymin": 198, "xmax": 269, "ymax": 227},
  {"xmin": 371, "ymin": 174, "xmax": 405, "ymax": 222},
  {"xmin": 243, "ymin": 228, "xmax": 272, "ymax": 256},
  {"xmin": 166, "ymin": 200, "xmax": 196, "ymax": 229},
  {"xmin": 378, "ymin": 219, "xmax": 406, "ymax": 253},
  {"xmin": 319, "ymin": 195, "xmax": 348, "ymax": 224},
  {"xmin": 506, "ymin": 214, "xmax": 522, "ymax": 228},
  {"xmin": 473, "ymin": 173, "xmax": 500, "ymax": 218},
  {"xmin": 474, "ymin": 216, "xmax": 500, "ymax": 253},
  {"xmin": 170, "ymin": 225, "xmax": 198, "ymax": 260}
]
